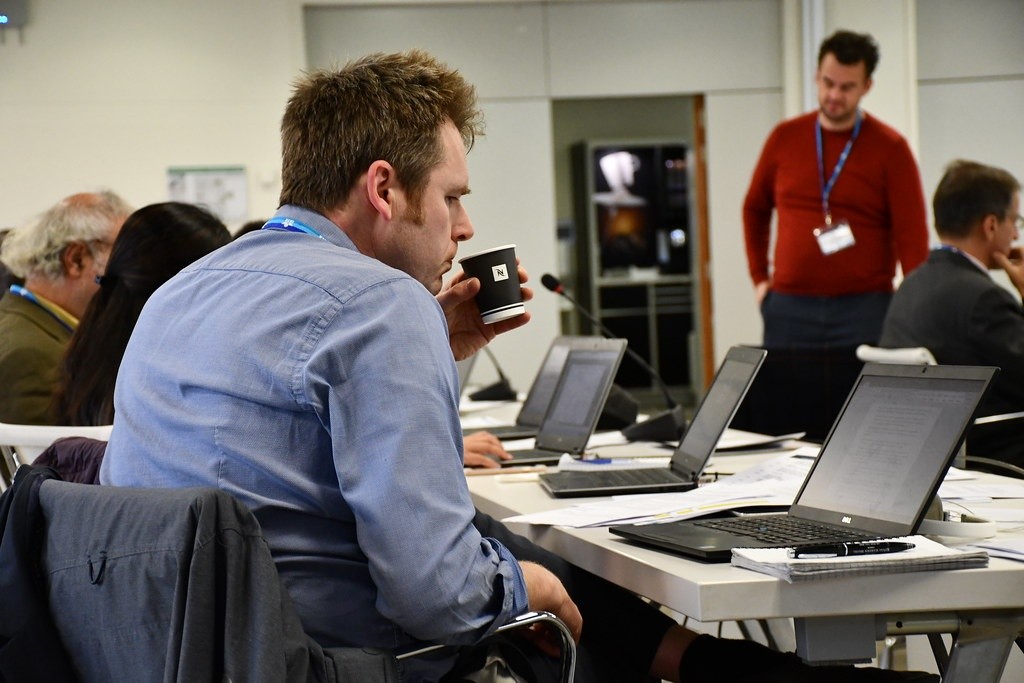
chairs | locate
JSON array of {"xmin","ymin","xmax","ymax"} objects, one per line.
[
  {"xmin": 0, "ymin": 422, "xmax": 115, "ymax": 494},
  {"xmin": 39, "ymin": 479, "xmax": 578, "ymax": 683},
  {"xmin": 855, "ymin": 344, "xmax": 1024, "ymax": 478}
]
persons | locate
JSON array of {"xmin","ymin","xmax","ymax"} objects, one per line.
[
  {"xmin": 868, "ymin": 158, "xmax": 1024, "ymax": 481},
  {"xmin": 44, "ymin": 200, "xmax": 233, "ymax": 425},
  {"xmin": 99, "ymin": 45, "xmax": 944, "ymax": 683},
  {"xmin": 727, "ymin": 28, "xmax": 931, "ymax": 444},
  {"xmin": 1, "ymin": 185, "xmax": 141, "ymax": 425}
]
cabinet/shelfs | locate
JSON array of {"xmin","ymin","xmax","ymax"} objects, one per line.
[{"xmin": 569, "ymin": 137, "xmax": 703, "ymax": 404}]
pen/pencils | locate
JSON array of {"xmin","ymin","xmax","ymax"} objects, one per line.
[
  {"xmin": 786, "ymin": 540, "xmax": 917, "ymax": 559},
  {"xmin": 573, "ymin": 457, "xmax": 612, "ymax": 463}
]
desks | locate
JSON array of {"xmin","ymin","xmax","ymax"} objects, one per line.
[{"xmin": 456, "ymin": 385, "xmax": 1024, "ymax": 683}]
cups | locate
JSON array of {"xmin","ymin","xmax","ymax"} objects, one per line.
[{"xmin": 457, "ymin": 245, "xmax": 526, "ymax": 324}]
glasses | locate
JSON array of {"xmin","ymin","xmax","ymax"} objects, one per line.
[{"xmin": 1003, "ymin": 211, "xmax": 1024, "ymax": 229}]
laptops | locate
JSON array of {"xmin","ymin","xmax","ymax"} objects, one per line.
[{"xmin": 457, "ymin": 331, "xmax": 1001, "ymax": 563}]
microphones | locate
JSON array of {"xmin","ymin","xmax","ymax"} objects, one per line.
[{"xmin": 539, "ymin": 271, "xmax": 688, "ymax": 442}]
